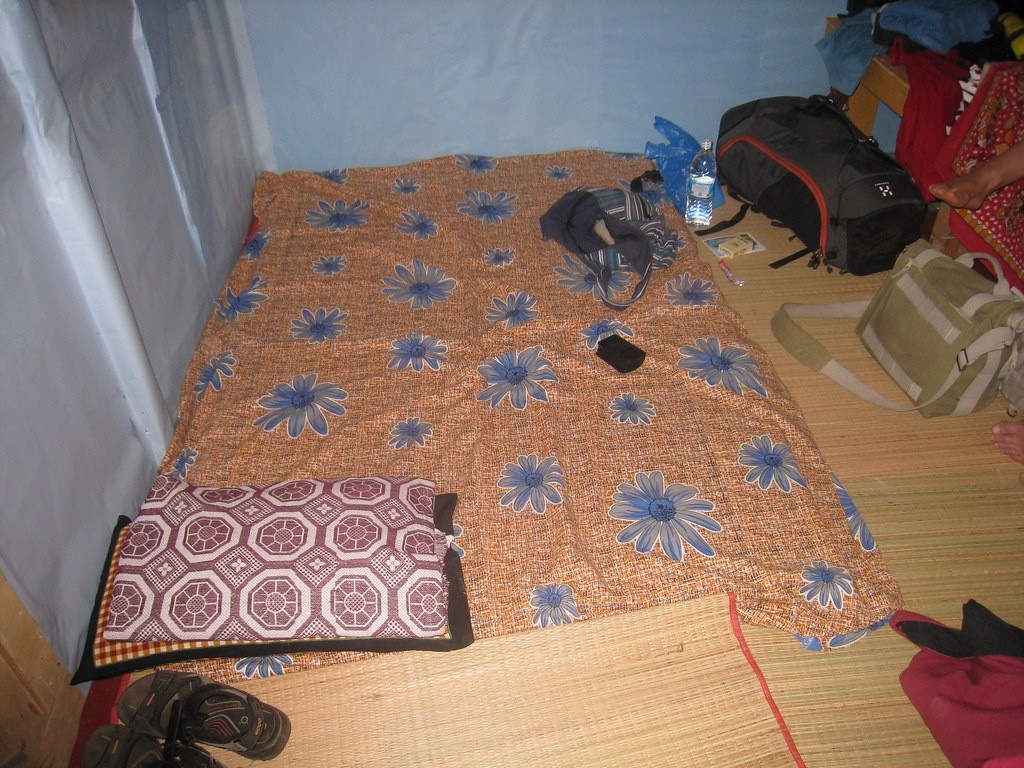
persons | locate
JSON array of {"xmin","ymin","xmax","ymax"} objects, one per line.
[
  {"xmin": 929, "ymin": 140, "xmax": 1024, "ymax": 209},
  {"xmin": 991, "ymin": 420, "xmax": 1024, "ymax": 488}
]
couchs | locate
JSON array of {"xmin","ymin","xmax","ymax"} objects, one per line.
[{"xmin": 929, "ymin": 63, "xmax": 1024, "ymax": 302}]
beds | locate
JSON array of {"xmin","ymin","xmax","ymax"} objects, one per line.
[{"xmin": 117, "ymin": 147, "xmax": 901, "ymax": 704}]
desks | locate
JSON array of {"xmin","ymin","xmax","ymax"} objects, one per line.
[{"xmin": 824, "ymin": 15, "xmax": 972, "ymax": 160}]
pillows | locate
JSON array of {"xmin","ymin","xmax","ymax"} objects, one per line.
[
  {"xmin": 69, "ymin": 493, "xmax": 475, "ymax": 686},
  {"xmin": 100, "ymin": 471, "xmax": 453, "ymax": 643}
]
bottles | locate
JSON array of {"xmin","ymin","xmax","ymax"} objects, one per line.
[{"xmin": 684, "ymin": 138, "xmax": 716, "ymax": 229}]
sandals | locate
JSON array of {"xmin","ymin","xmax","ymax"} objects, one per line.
[
  {"xmin": 118, "ymin": 670, "xmax": 291, "ymax": 760},
  {"xmin": 80, "ymin": 725, "xmax": 227, "ymax": 768}
]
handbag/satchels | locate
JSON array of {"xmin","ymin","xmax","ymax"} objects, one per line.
[
  {"xmin": 644, "ymin": 115, "xmax": 724, "ymax": 217},
  {"xmin": 771, "ymin": 238, "xmax": 1024, "ymax": 419},
  {"xmin": 695, "ymin": 87, "xmax": 927, "ymax": 276}
]
